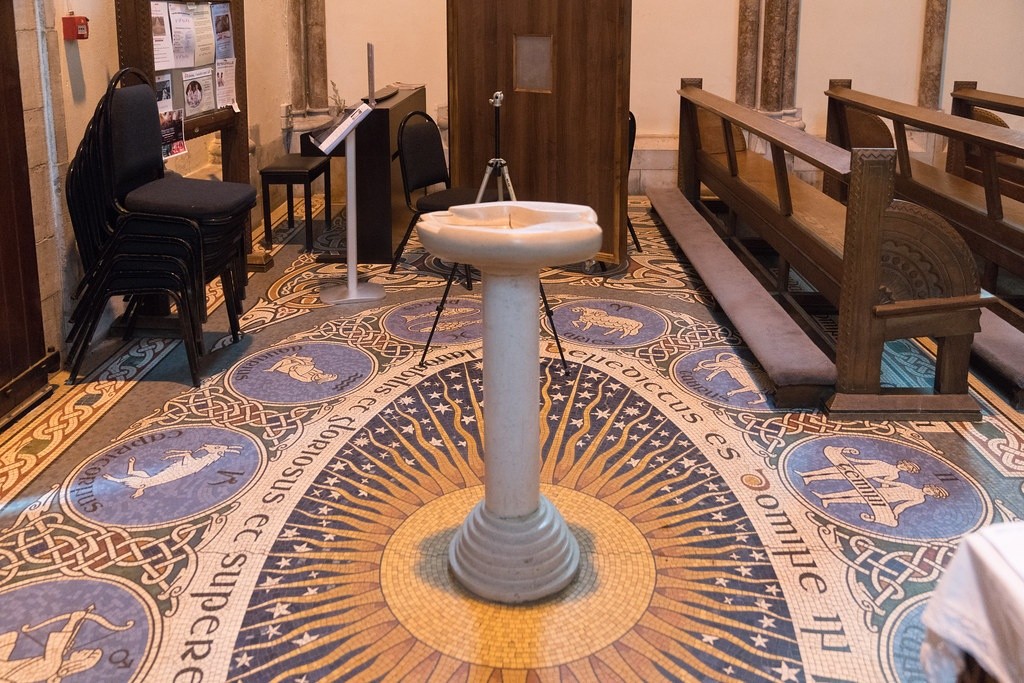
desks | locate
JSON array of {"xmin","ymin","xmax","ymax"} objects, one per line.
[{"xmin": 921, "ymin": 521, "xmax": 1024, "ymax": 683}]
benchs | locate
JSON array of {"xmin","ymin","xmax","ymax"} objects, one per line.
[{"xmin": 645, "ymin": 77, "xmax": 1024, "ymax": 423}]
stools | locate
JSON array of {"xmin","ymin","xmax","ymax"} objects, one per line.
[{"xmin": 259, "ymin": 153, "xmax": 331, "ymax": 251}]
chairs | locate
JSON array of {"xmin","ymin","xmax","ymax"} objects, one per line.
[
  {"xmin": 627, "ymin": 110, "xmax": 642, "ymax": 255},
  {"xmin": 390, "ymin": 110, "xmax": 474, "ymax": 290},
  {"xmin": 64, "ymin": 66, "xmax": 257, "ymax": 387}
]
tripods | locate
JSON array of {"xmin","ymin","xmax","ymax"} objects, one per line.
[{"xmin": 420, "ymin": 93, "xmax": 571, "ymax": 377}]
